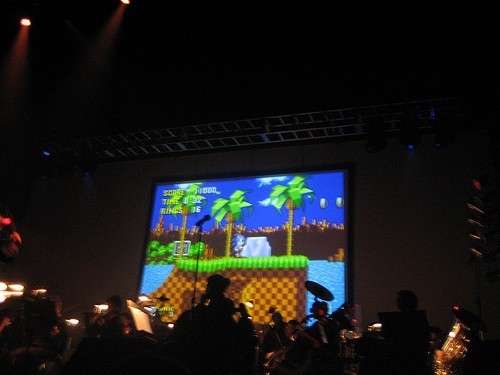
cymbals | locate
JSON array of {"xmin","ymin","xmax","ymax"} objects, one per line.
[{"xmin": 304, "ymin": 280, "xmax": 333, "ymax": 301}]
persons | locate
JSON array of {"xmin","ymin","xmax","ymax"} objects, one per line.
[{"xmin": 0, "ymin": 275, "xmax": 442, "ymax": 375}]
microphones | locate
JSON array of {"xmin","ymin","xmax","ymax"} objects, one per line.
[{"xmin": 197, "ymin": 214, "xmax": 211, "ymax": 227}]
